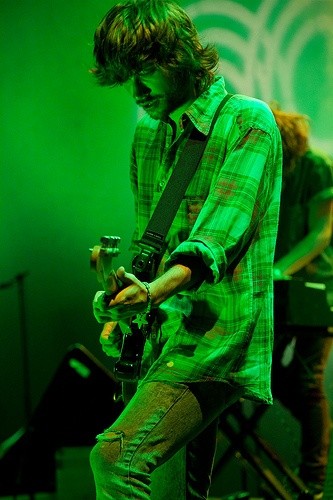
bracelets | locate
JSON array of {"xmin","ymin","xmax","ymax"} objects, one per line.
[{"xmin": 132, "ymin": 282, "xmax": 152, "ymax": 329}]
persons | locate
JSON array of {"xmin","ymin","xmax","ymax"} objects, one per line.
[
  {"xmin": 86, "ymin": 1, "xmax": 285, "ymax": 500},
  {"xmin": 266, "ymin": 104, "xmax": 333, "ymax": 493}
]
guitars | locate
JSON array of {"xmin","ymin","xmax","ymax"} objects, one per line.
[{"xmin": 87, "ymin": 235, "xmax": 162, "ymax": 383}]
